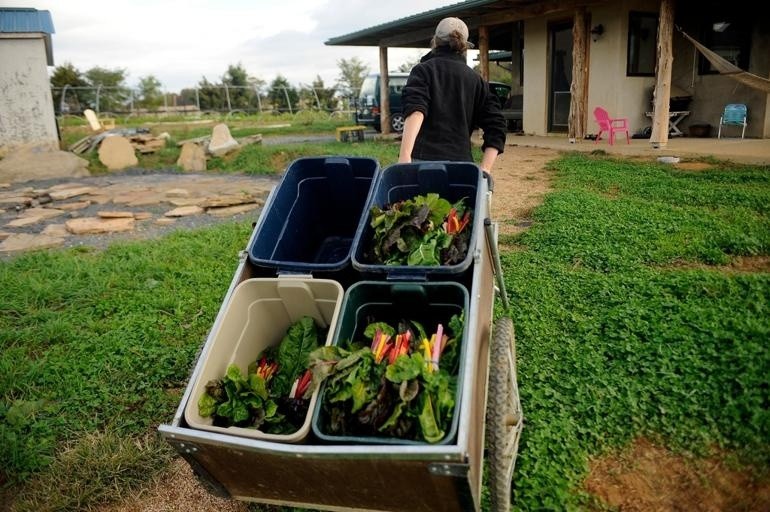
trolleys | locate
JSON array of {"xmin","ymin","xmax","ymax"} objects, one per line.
[{"xmin": 155, "ymin": 156, "xmax": 525, "ymax": 511}]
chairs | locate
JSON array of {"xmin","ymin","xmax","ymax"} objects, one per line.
[
  {"xmin": 717, "ymin": 103, "xmax": 748, "ymax": 140},
  {"xmin": 593, "ymin": 106, "xmax": 630, "ymax": 146}
]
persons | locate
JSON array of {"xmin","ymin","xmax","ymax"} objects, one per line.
[{"xmin": 398, "ymin": 15, "xmax": 508, "ymax": 177}]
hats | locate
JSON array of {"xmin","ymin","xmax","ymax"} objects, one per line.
[{"xmin": 436, "ymin": 17, "xmax": 469, "ymax": 45}]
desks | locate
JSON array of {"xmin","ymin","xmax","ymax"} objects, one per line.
[{"xmin": 645, "ymin": 110, "xmax": 692, "ymax": 138}]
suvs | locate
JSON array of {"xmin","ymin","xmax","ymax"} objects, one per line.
[{"xmin": 355, "ymin": 72, "xmax": 411, "ymax": 133}]
